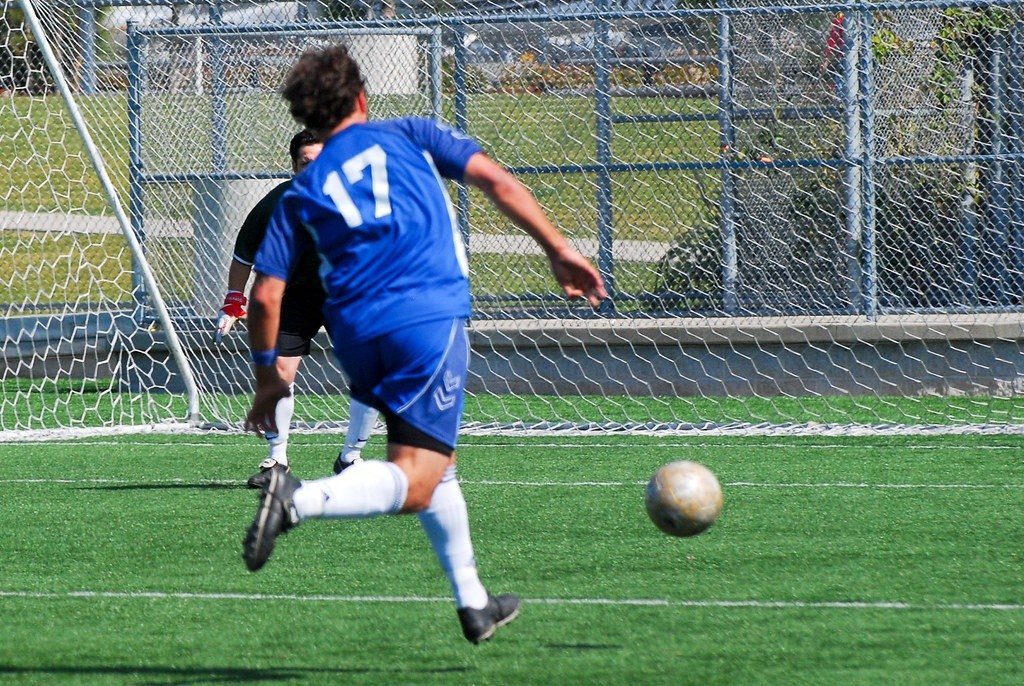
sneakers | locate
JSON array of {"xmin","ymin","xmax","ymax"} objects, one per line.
[
  {"xmin": 244, "ymin": 468, "xmax": 301, "ymax": 571},
  {"xmin": 457, "ymin": 591, "xmax": 519, "ymax": 643},
  {"xmin": 248, "ymin": 458, "xmax": 291, "ymax": 486},
  {"xmin": 334, "ymin": 453, "xmax": 364, "ymax": 474}
]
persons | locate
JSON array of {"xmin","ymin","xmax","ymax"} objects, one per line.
[
  {"xmin": 240, "ymin": 43, "xmax": 608, "ymax": 643},
  {"xmin": 212, "ymin": 126, "xmax": 380, "ymax": 488}
]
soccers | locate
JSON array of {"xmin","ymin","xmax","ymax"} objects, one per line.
[{"xmin": 644, "ymin": 458, "xmax": 725, "ymax": 537}]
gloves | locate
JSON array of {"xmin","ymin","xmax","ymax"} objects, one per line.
[{"xmin": 212, "ymin": 291, "xmax": 246, "ymax": 344}]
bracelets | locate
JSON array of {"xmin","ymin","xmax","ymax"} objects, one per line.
[{"xmin": 249, "ymin": 348, "xmax": 278, "ymax": 367}]
